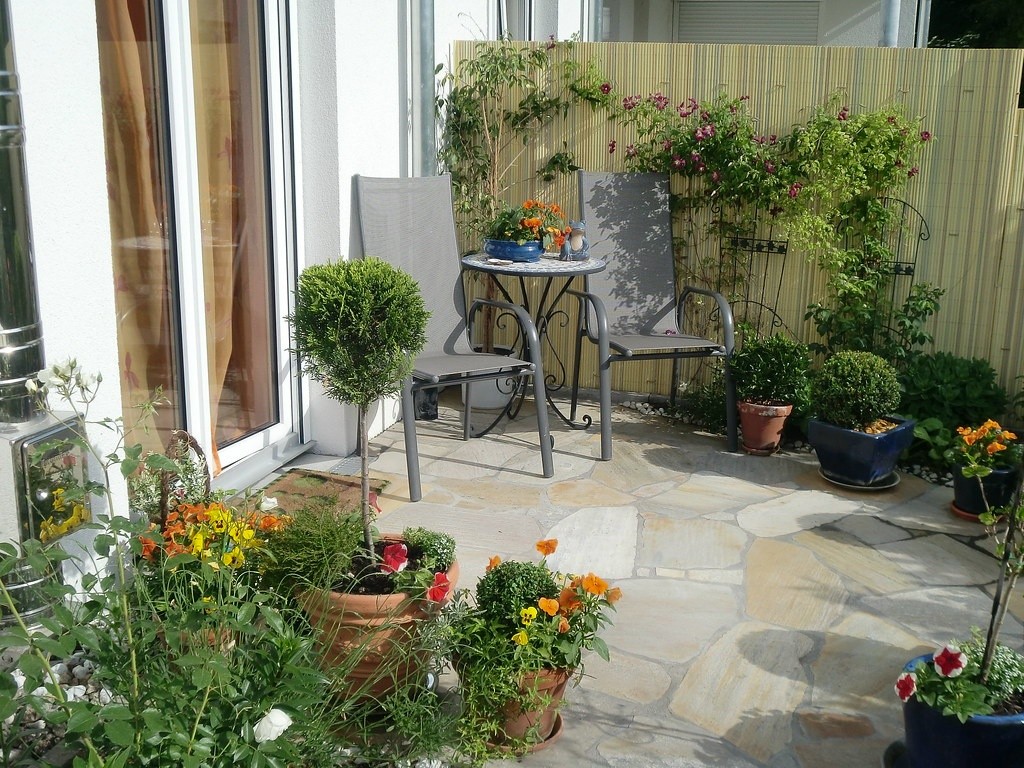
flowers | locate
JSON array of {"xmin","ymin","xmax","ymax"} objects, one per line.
[
  {"xmin": 893, "ymin": 479, "xmax": 1024, "ymax": 723},
  {"xmin": 483, "ymin": 201, "xmax": 571, "ymax": 247},
  {"xmin": 945, "ymin": 416, "xmax": 1019, "ymax": 471},
  {"xmin": 381, "ymin": 539, "xmax": 622, "ymax": 690}
]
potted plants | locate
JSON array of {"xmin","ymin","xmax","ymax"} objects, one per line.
[
  {"xmin": 728, "ymin": 331, "xmax": 809, "ymax": 456},
  {"xmin": 433, "ymin": 28, "xmax": 584, "ymax": 409},
  {"xmin": 810, "ymin": 349, "xmax": 918, "ymax": 490}
]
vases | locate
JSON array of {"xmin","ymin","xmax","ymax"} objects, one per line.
[
  {"xmin": 882, "ymin": 653, "xmax": 1024, "ymax": 768},
  {"xmin": 951, "ymin": 468, "xmax": 1019, "ymax": 525},
  {"xmin": 450, "ymin": 651, "xmax": 576, "ymax": 756},
  {"xmin": 284, "ymin": 254, "xmax": 459, "ymax": 699},
  {"xmin": 485, "ymin": 240, "xmax": 540, "ymax": 261}
]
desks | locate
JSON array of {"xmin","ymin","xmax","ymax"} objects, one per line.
[{"xmin": 462, "ymin": 253, "xmax": 606, "ymax": 448}]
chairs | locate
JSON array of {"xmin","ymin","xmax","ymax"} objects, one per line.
[
  {"xmin": 570, "ymin": 170, "xmax": 738, "ymax": 461},
  {"xmin": 356, "ymin": 172, "xmax": 554, "ymax": 502}
]
